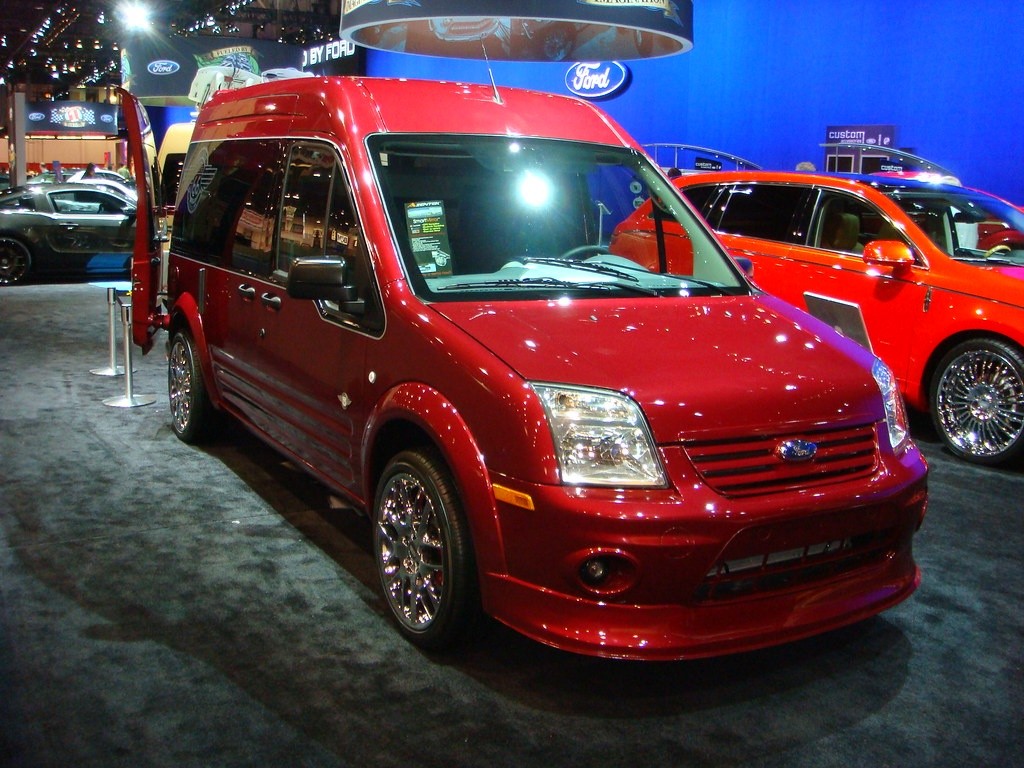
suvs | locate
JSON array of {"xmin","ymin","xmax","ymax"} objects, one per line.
[{"xmin": 608, "ymin": 173, "xmax": 1024, "ymax": 461}]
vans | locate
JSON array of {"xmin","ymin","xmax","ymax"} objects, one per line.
[
  {"xmin": 157, "ymin": 123, "xmax": 197, "ymax": 252},
  {"xmin": 111, "ymin": 75, "xmax": 930, "ymax": 664}
]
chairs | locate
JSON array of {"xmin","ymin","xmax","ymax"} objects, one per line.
[{"xmin": 831, "ymin": 213, "xmax": 864, "ymax": 254}]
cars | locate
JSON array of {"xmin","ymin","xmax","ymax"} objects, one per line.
[{"xmin": 0, "ymin": 169, "xmax": 138, "ymax": 286}]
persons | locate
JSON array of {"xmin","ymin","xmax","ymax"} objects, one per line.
[
  {"xmin": 117, "ymin": 162, "xmax": 130, "ymax": 179},
  {"xmin": 82, "ymin": 162, "xmax": 95, "ymax": 179}
]
